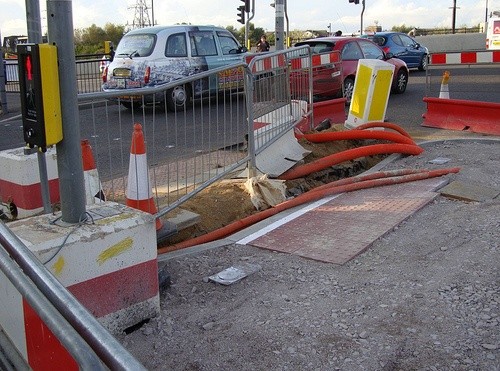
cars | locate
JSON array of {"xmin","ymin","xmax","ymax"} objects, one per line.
[
  {"xmin": 349, "ymin": 32, "xmax": 430, "ymax": 72},
  {"xmin": 100, "ymin": 24, "xmax": 244, "ymax": 113},
  {"xmin": 287, "ymin": 36, "xmax": 410, "ymax": 107}
]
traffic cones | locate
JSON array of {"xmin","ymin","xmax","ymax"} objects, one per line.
[
  {"xmin": 124, "ymin": 122, "xmax": 164, "ymax": 232},
  {"xmin": 439, "ymin": 70, "xmax": 450, "ymax": 99},
  {"xmin": 81, "ymin": 138, "xmax": 106, "ymax": 203}
]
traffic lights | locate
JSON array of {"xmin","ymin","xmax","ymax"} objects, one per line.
[
  {"xmin": 349, "ymin": 0, "xmax": 360, "ymax": 5},
  {"xmin": 236, "ymin": 5, "xmax": 245, "ymax": 25},
  {"xmin": 240, "ymin": 0, "xmax": 250, "ymax": 13}
]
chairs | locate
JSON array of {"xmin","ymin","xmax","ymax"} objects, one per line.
[
  {"xmin": 169, "ymin": 37, "xmax": 185, "ymax": 56},
  {"xmin": 199, "ymin": 37, "xmax": 217, "ymax": 54}
]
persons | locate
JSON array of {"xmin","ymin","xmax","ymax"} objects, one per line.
[
  {"xmin": 257, "ymin": 35, "xmax": 270, "ymax": 52},
  {"xmin": 408, "ymin": 28, "xmax": 419, "ymax": 37},
  {"xmin": 335, "ymin": 30, "xmax": 342, "ymax": 37}
]
